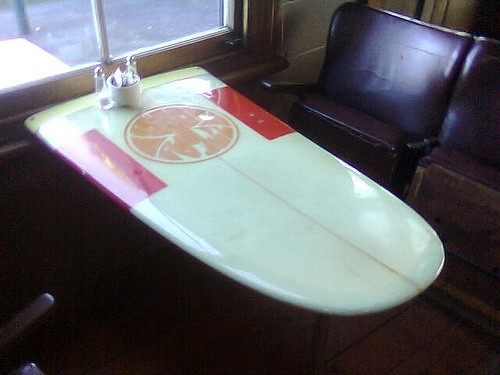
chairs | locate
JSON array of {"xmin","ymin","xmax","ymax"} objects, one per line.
[
  {"xmin": 403, "ymin": 36, "xmax": 499, "ymax": 280},
  {"xmin": 0, "ymin": 293, "xmax": 55, "ymax": 375},
  {"xmin": 259, "ymin": 1, "xmax": 474, "ymax": 192}
]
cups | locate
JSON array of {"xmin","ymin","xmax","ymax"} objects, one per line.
[{"xmin": 106, "ymin": 73, "xmax": 140, "ymax": 107}]
